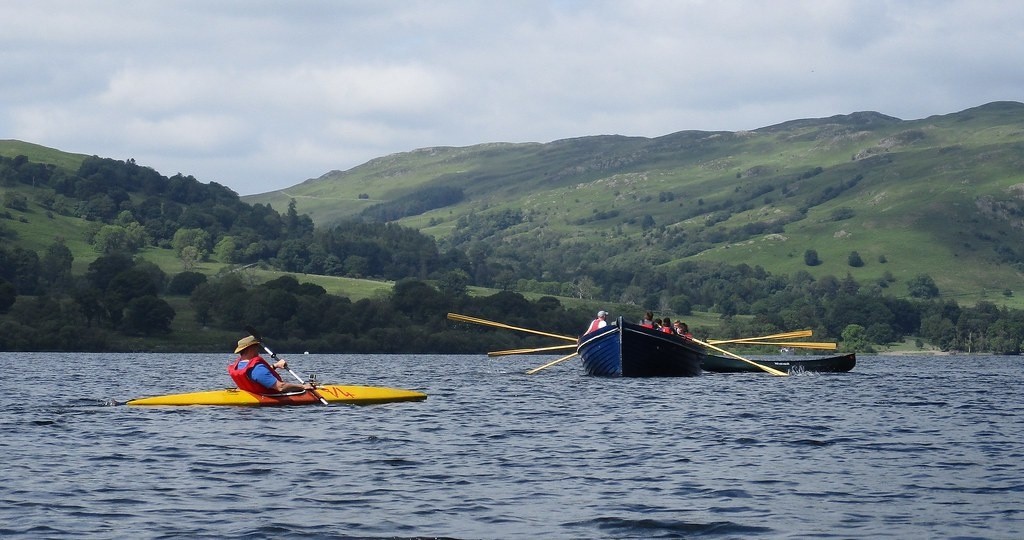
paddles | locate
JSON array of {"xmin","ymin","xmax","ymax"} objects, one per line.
[
  {"xmin": 247, "ymin": 327, "xmax": 331, "ymax": 407},
  {"xmin": 447, "ymin": 313, "xmax": 578, "ymax": 341},
  {"xmin": 704, "ymin": 340, "xmax": 837, "ymax": 350},
  {"xmin": 529, "ymin": 352, "xmax": 578, "ymax": 374},
  {"xmin": 706, "ymin": 330, "xmax": 812, "ymax": 345},
  {"xmin": 488, "ymin": 344, "xmax": 577, "ymax": 356},
  {"xmin": 656, "ymin": 324, "xmax": 789, "ymax": 376}
]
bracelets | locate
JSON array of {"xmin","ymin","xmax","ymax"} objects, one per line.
[{"xmin": 271, "ymin": 364, "xmax": 276, "ymax": 369}]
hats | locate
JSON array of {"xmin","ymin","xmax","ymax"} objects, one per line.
[
  {"xmin": 234, "ymin": 335, "xmax": 260, "ymax": 353},
  {"xmin": 597, "ymin": 311, "xmax": 609, "ymax": 317}
]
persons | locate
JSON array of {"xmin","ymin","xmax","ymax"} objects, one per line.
[
  {"xmin": 638, "ymin": 311, "xmax": 693, "ymax": 341},
  {"xmin": 582, "ymin": 311, "xmax": 607, "ymax": 336},
  {"xmin": 234, "ymin": 335, "xmax": 316, "ymax": 393}
]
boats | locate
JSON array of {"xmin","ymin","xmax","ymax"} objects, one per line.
[
  {"xmin": 577, "ymin": 315, "xmax": 707, "ymax": 378},
  {"xmin": 125, "ymin": 384, "xmax": 428, "ymax": 407},
  {"xmin": 700, "ymin": 352, "xmax": 856, "ymax": 374}
]
